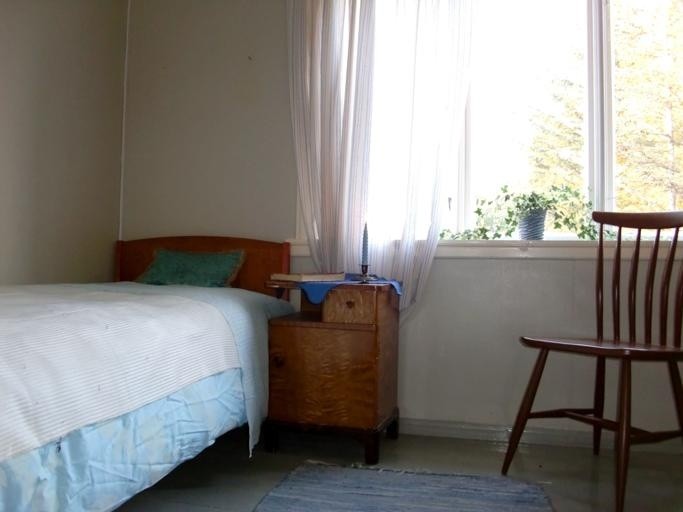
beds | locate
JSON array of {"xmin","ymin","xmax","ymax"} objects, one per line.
[{"xmin": 0, "ymin": 235, "xmax": 291, "ymax": 512}]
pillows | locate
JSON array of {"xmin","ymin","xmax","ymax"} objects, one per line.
[{"xmin": 133, "ymin": 248, "xmax": 246, "ymax": 288}]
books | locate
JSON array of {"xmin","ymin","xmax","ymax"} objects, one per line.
[{"xmin": 270, "ymin": 271, "xmax": 345, "ymax": 282}]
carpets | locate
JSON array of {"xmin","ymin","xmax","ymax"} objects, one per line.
[{"xmin": 252, "ymin": 460, "xmax": 555, "ymax": 512}]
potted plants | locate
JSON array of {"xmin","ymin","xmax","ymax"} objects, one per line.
[{"xmin": 439, "ymin": 184, "xmax": 617, "ymax": 241}]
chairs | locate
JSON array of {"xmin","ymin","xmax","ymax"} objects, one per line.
[{"xmin": 501, "ymin": 210, "xmax": 683, "ymax": 512}]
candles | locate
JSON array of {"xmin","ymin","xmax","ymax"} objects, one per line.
[{"xmin": 361, "ymin": 222, "xmax": 369, "ymax": 265}]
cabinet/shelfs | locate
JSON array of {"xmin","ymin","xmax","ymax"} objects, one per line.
[{"xmin": 263, "ymin": 278, "xmax": 404, "ymax": 465}]
